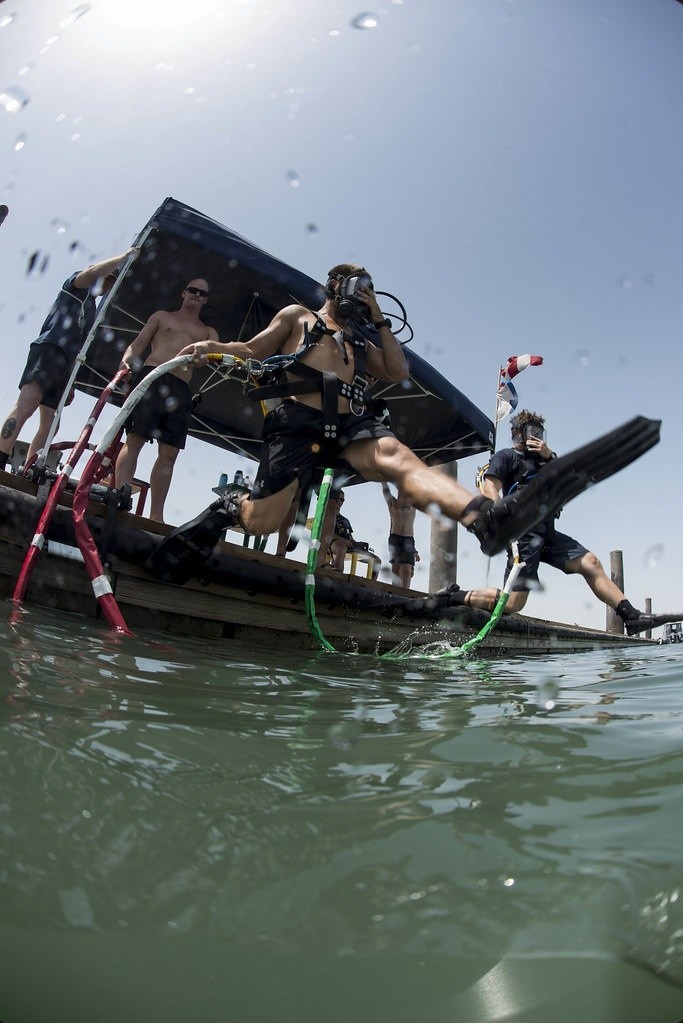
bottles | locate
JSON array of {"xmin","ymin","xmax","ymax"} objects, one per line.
[
  {"xmin": 233, "ymin": 470, "xmax": 243, "ymax": 485},
  {"xmin": 242, "ymin": 476, "xmax": 254, "ymax": 490},
  {"xmin": 218, "ymin": 474, "xmax": 228, "ymax": 486}
]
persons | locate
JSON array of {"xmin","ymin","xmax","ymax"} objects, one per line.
[
  {"xmin": 381, "ymin": 481, "xmax": 420, "ymax": 589},
  {"xmin": 114, "ymin": 276, "xmax": 220, "ymax": 523},
  {"xmin": 329, "ymin": 490, "xmax": 354, "ymax": 573},
  {"xmin": 409, "ymin": 412, "xmax": 683, "ymax": 636},
  {"xmin": 273, "ymin": 487, "xmax": 341, "ymax": 571},
  {"xmin": 147, "ymin": 263, "xmax": 662, "ymax": 587},
  {"xmin": 0, "ymin": 246, "xmax": 140, "ymax": 471}
]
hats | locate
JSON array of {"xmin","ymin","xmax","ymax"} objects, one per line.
[{"xmin": 110, "ymin": 268, "xmax": 120, "ymax": 279}]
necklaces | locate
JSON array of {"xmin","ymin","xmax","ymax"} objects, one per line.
[{"xmin": 328, "ymin": 316, "xmax": 342, "ymax": 330}]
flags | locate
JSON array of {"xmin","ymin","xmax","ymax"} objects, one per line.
[
  {"xmin": 496, "ymin": 381, "xmax": 518, "ymax": 422},
  {"xmin": 501, "ymin": 354, "xmax": 543, "ymax": 381}
]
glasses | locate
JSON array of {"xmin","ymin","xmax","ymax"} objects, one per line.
[
  {"xmin": 184, "ymin": 286, "xmax": 209, "ymax": 297},
  {"xmin": 339, "ymin": 498, "xmax": 345, "ymax": 502}
]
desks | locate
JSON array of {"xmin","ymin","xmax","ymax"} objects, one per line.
[
  {"xmin": 100, "ymin": 443, "xmax": 151, "ymax": 517},
  {"xmin": 213, "ymin": 483, "xmax": 268, "ymax": 551}
]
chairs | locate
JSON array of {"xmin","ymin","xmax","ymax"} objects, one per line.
[{"xmin": 306, "ymin": 517, "xmax": 375, "ymax": 580}]
